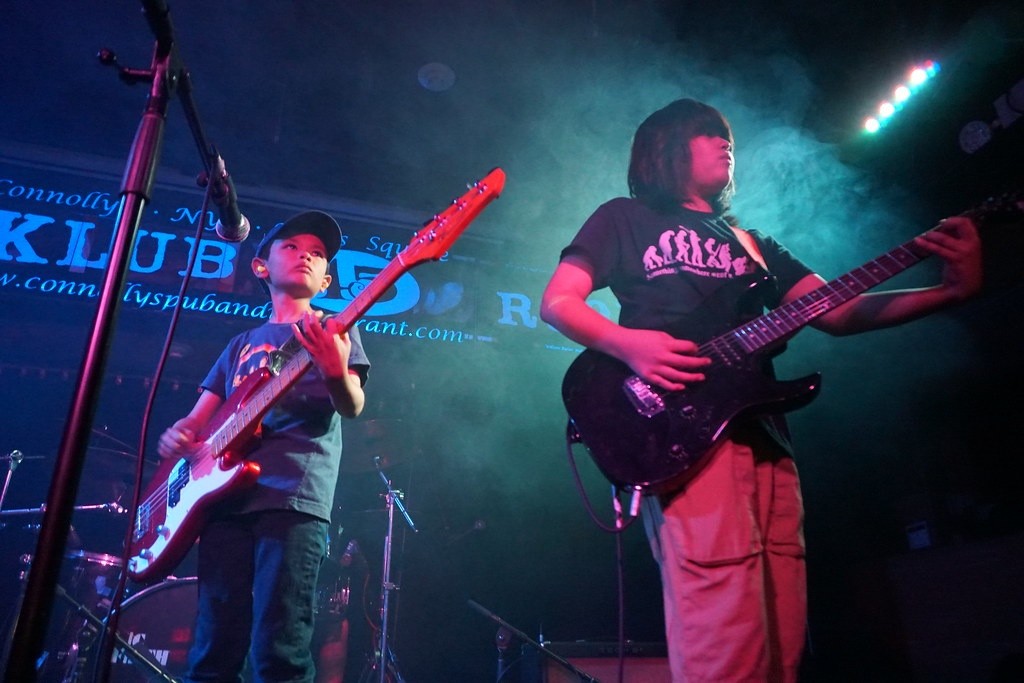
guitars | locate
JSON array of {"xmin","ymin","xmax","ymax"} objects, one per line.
[
  {"xmin": 561, "ymin": 189, "xmax": 1023, "ymax": 495},
  {"xmin": 123, "ymin": 165, "xmax": 507, "ymax": 582}
]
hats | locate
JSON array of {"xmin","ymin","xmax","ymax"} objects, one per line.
[{"xmin": 256, "ymin": 212, "xmax": 341, "ymax": 293}]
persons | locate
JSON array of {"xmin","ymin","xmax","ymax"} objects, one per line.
[
  {"xmin": 157, "ymin": 209, "xmax": 371, "ymax": 683},
  {"xmin": 539, "ymin": 97, "xmax": 984, "ymax": 682}
]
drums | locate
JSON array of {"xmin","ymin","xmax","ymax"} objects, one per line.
[
  {"xmin": 39, "ymin": 549, "xmax": 124, "ymax": 667},
  {"xmin": 79, "ymin": 574, "xmax": 201, "ymax": 683}
]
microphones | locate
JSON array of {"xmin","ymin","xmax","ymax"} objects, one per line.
[
  {"xmin": 339, "ymin": 540, "xmax": 357, "ymax": 569},
  {"xmin": 210, "ymin": 152, "xmax": 252, "ymax": 245}
]
